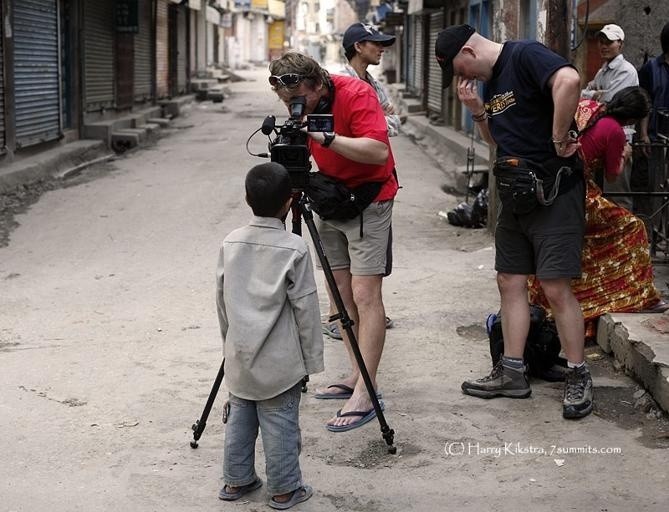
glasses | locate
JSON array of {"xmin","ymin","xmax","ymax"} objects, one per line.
[{"xmin": 268, "ymin": 73, "xmax": 306, "ymax": 88}]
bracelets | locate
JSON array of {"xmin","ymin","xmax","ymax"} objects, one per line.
[
  {"xmin": 621, "ymin": 155, "xmax": 626, "ymax": 162},
  {"xmin": 552, "ymin": 137, "xmax": 569, "ymax": 143},
  {"xmin": 472, "ymin": 109, "xmax": 487, "ymax": 121}
]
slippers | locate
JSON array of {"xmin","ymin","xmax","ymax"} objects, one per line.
[
  {"xmin": 325, "ymin": 400, "xmax": 387, "ymax": 431},
  {"xmin": 219, "ymin": 479, "xmax": 263, "ymax": 498},
  {"xmin": 265, "ymin": 485, "xmax": 314, "ymax": 511},
  {"xmin": 314, "ymin": 383, "xmax": 384, "ymax": 401}
]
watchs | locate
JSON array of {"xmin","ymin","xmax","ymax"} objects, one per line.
[{"xmin": 321, "ymin": 130, "xmax": 335, "ymax": 147}]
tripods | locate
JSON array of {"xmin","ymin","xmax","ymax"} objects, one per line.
[{"xmin": 189, "ymin": 193, "xmax": 398, "ymax": 455}]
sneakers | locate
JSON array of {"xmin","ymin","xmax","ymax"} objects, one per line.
[
  {"xmin": 320, "ymin": 312, "xmax": 394, "ymax": 342},
  {"xmin": 461, "ymin": 356, "xmax": 532, "ymax": 400},
  {"xmin": 561, "ymin": 363, "xmax": 596, "ymax": 421}
]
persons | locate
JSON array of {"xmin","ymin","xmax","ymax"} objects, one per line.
[
  {"xmin": 582, "ymin": 24, "xmax": 640, "ymax": 211},
  {"xmin": 636, "ymin": 22, "xmax": 669, "ymax": 190},
  {"xmin": 435, "ymin": 24, "xmax": 594, "ymax": 418},
  {"xmin": 322, "ymin": 23, "xmax": 400, "ymax": 339},
  {"xmin": 215, "ymin": 162, "xmax": 325, "ymax": 507},
  {"xmin": 269, "ymin": 54, "xmax": 398, "ymax": 433},
  {"xmin": 524, "ymin": 86, "xmax": 669, "ymax": 336}
]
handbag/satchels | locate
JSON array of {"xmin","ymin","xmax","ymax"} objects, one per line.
[
  {"xmin": 482, "ymin": 301, "xmax": 563, "ymax": 380},
  {"xmin": 489, "ymin": 153, "xmax": 573, "ymax": 215}
]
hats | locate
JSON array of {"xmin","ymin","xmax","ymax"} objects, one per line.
[
  {"xmin": 342, "ymin": 22, "xmax": 396, "ymax": 50},
  {"xmin": 434, "ymin": 23, "xmax": 477, "ymax": 89},
  {"xmin": 595, "ymin": 23, "xmax": 626, "ymax": 43}
]
